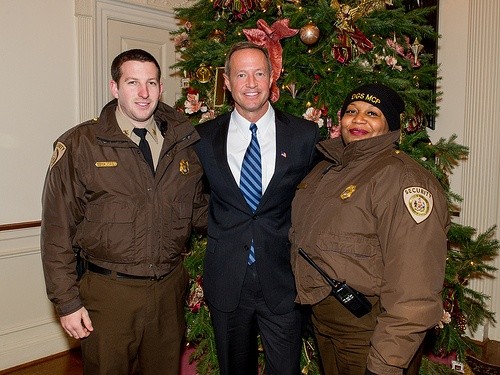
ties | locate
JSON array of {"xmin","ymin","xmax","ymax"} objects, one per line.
[
  {"xmin": 131, "ymin": 127, "xmax": 155, "ymax": 180},
  {"xmin": 235, "ymin": 123, "xmax": 263, "ymax": 265}
]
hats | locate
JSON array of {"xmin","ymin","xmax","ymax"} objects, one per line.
[{"xmin": 340, "ymin": 84, "xmax": 405, "ymax": 131}]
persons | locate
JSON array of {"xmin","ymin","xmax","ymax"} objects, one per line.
[
  {"xmin": 289, "ymin": 84, "xmax": 447, "ymax": 375},
  {"xmin": 193, "ymin": 44, "xmax": 324, "ymax": 375},
  {"xmin": 40, "ymin": 49, "xmax": 210, "ymax": 375}
]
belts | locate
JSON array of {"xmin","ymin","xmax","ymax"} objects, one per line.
[{"xmin": 80, "ymin": 258, "xmax": 175, "ymax": 281}]
